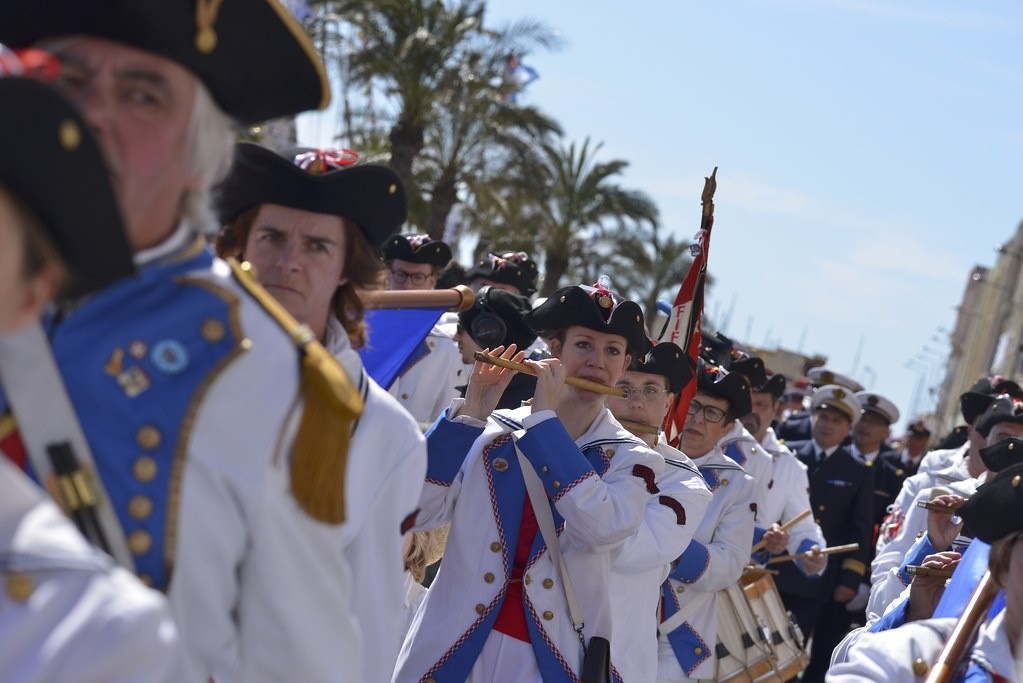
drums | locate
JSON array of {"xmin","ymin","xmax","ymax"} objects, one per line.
[
  {"xmin": 715, "ymin": 580, "xmax": 778, "ymax": 683},
  {"xmin": 739, "ymin": 568, "xmax": 810, "ymax": 683}
]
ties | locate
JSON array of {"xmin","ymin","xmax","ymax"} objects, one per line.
[{"xmin": 818, "ymin": 451, "xmax": 826, "ymax": 463}]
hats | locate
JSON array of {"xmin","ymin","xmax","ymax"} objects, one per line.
[
  {"xmin": 234, "ymin": 139, "xmax": 407, "ymax": 244},
  {"xmin": 959, "ymin": 374, "xmax": 1023, "ymax": 544},
  {"xmin": 0, "ymin": 74, "xmax": 140, "ymax": 306},
  {"xmin": 0, "ymin": 0, "xmax": 334, "ymax": 127},
  {"xmin": 785, "ymin": 369, "xmax": 932, "ymax": 439},
  {"xmin": 385, "ymin": 231, "xmax": 785, "ymax": 418}
]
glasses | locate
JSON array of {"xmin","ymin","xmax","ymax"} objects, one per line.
[
  {"xmin": 687, "ymin": 399, "xmax": 727, "ymax": 423},
  {"xmin": 613, "ymin": 384, "xmax": 669, "ymax": 401},
  {"xmin": 457, "ymin": 322, "xmax": 468, "ymax": 334},
  {"xmin": 388, "ymin": 268, "xmax": 434, "ymax": 286}
]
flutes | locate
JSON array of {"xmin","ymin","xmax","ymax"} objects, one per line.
[
  {"xmin": 473, "ymin": 351, "xmax": 627, "ymax": 398},
  {"xmin": 917, "ymin": 500, "xmax": 959, "ymax": 514},
  {"xmin": 521, "ymin": 400, "xmax": 661, "ymax": 435},
  {"xmin": 904, "ymin": 565, "xmax": 955, "ymax": 578}
]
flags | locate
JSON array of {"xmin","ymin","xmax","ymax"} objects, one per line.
[{"xmin": 657, "ymin": 224, "xmax": 711, "ymax": 360}]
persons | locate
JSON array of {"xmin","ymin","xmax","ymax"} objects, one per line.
[
  {"xmin": 658, "ymin": 367, "xmax": 757, "ymax": 682},
  {"xmin": 391, "ymin": 274, "xmax": 667, "ymax": 682},
  {"xmin": 215, "ymin": 142, "xmax": 427, "ymax": 682},
  {"xmin": 700, "ymin": 334, "xmax": 1023, "ymax": 683},
  {"xmin": 360, "ymin": 231, "xmax": 555, "ymax": 630},
  {"xmin": 1, "ymin": 1, "xmax": 362, "ymax": 683},
  {"xmin": 606, "ymin": 339, "xmax": 715, "ymax": 683},
  {"xmin": 0, "ymin": 47, "xmax": 206, "ymax": 682}
]
